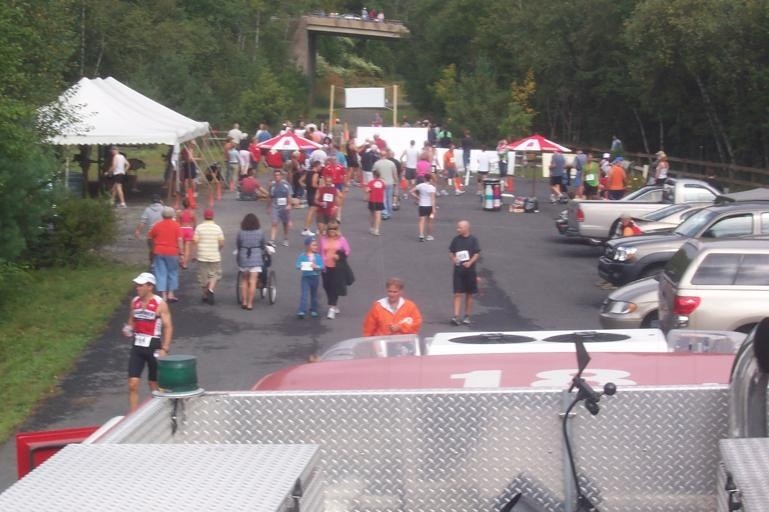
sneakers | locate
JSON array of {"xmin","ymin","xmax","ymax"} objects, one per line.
[
  {"xmin": 301, "ymin": 229, "xmax": 315, "ymax": 236},
  {"xmin": 452, "ymin": 316, "xmax": 462, "ymax": 325},
  {"xmin": 456, "ymin": 190, "xmax": 465, "ymax": 196},
  {"xmin": 168, "ymin": 297, "xmax": 179, "ymax": 303},
  {"xmin": 117, "ymin": 203, "xmax": 127, "ymax": 209},
  {"xmin": 282, "ymin": 239, "xmax": 289, "ymax": 247},
  {"xmin": 462, "ymin": 316, "xmax": 472, "ymax": 323},
  {"xmin": 426, "ymin": 236, "xmax": 433, "ymax": 241},
  {"xmin": 268, "ymin": 241, "xmax": 277, "ymax": 248},
  {"xmin": 203, "ymin": 293, "xmax": 208, "ymax": 302},
  {"xmin": 296, "ymin": 306, "xmax": 340, "ymax": 320},
  {"xmin": 207, "ymin": 288, "xmax": 215, "ymax": 305},
  {"xmin": 419, "ymin": 235, "xmax": 424, "ymax": 242}
]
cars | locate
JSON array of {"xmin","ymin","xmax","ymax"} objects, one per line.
[{"xmin": 555, "ymin": 175, "xmax": 768, "ymax": 327}]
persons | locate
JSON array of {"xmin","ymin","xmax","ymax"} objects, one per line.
[
  {"xmin": 122, "ymin": 272, "xmax": 172, "ymax": 415},
  {"xmin": 133, "ymin": 193, "xmax": 225, "ymax": 305},
  {"xmin": 447, "ymin": 220, "xmax": 481, "ymax": 326},
  {"xmin": 476, "ymin": 135, "xmax": 670, "ymax": 209},
  {"xmin": 346, "ymin": 114, "xmax": 464, "ymax": 242},
  {"xmin": 365, "ymin": 277, "xmax": 422, "ymax": 336},
  {"xmin": 206, "ymin": 119, "xmax": 355, "ymax": 320},
  {"xmin": 106, "ymin": 119, "xmax": 198, "ymax": 210}
]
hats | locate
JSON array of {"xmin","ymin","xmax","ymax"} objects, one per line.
[
  {"xmin": 611, "ymin": 157, "xmax": 624, "ymax": 164},
  {"xmin": 656, "ymin": 150, "xmax": 664, "ymax": 157},
  {"xmin": 203, "ymin": 209, "xmax": 214, "ymax": 218},
  {"xmin": 111, "ymin": 146, "xmax": 119, "ymax": 150},
  {"xmin": 163, "ymin": 207, "xmax": 173, "ymax": 219},
  {"xmin": 151, "ymin": 193, "xmax": 160, "ymax": 201},
  {"xmin": 132, "ymin": 272, "xmax": 157, "ymax": 286}
]
